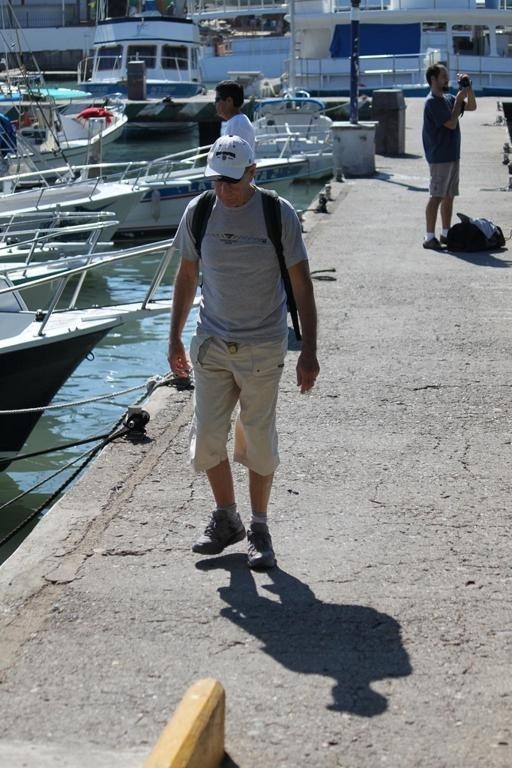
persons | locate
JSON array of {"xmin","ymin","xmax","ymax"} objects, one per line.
[
  {"xmin": 421, "ymin": 64, "xmax": 477, "ymax": 250},
  {"xmin": 167, "ymin": 134, "xmax": 321, "ymax": 568},
  {"xmin": 214, "ymin": 79, "xmax": 256, "ymax": 185}
]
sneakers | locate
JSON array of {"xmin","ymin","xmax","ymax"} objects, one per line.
[
  {"xmin": 422, "ymin": 238, "xmax": 447, "ymax": 250},
  {"xmin": 439, "ymin": 234, "xmax": 447, "ymax": 243},
  {"xmin": 191, "ymin": 510, "xmax": 246, "ymax": 555},
  {"xmin": 245, "ymin": 528, "xmax": 277, "ymax": 570}
]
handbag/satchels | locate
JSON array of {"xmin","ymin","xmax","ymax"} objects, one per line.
[{"xmin": 447, "ymin": 213, "xmax": 506, "ymax": 253}]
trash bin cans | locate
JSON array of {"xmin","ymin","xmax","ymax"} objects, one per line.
[
  {"xmin": 128, "ymin": 60, "xmax": 147, "ymax": 100},
  {"xmin": 372, "ymin": 88, "xmax": 407, "ymax": 156}
]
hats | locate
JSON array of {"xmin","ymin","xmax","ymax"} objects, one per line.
[{"xmin": 204, "ymin": 134, "xmax": 256, "ymax": 179}]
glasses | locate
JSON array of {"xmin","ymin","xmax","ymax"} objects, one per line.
[
  {"xmin": 212, "ymin": 175, "xmax": 241, "ymax": 184},
  {"xmin": 214, "ymin": 96, "xmax": 223, "ymax": 102}
]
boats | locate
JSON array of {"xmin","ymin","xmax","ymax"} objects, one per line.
[
  {"xmin": 1, "ymin": 1, "xmax": 205, "ymax": 471},
  {"xmin": 75, "ymin": 1, "xmax": 217, "ymax": 132},
  {"xmin": 281, "ymin": 0, "xmax": 512, "ymax": 96},
  {"xmin": 70, "ymin": 133, "xmax": 309, "ymax": 239},
  {"xmin": 247, "ymin": 96, "xmax": 339, "ymax": 182},
  {"xmin": 183, "ymin": 1, "xmax": 292, "ymax": 99}
]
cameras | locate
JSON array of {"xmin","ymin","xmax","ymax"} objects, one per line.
[{"xmin": 457, "ymin": 77, "xmax": 472, "ymax": 88}]
